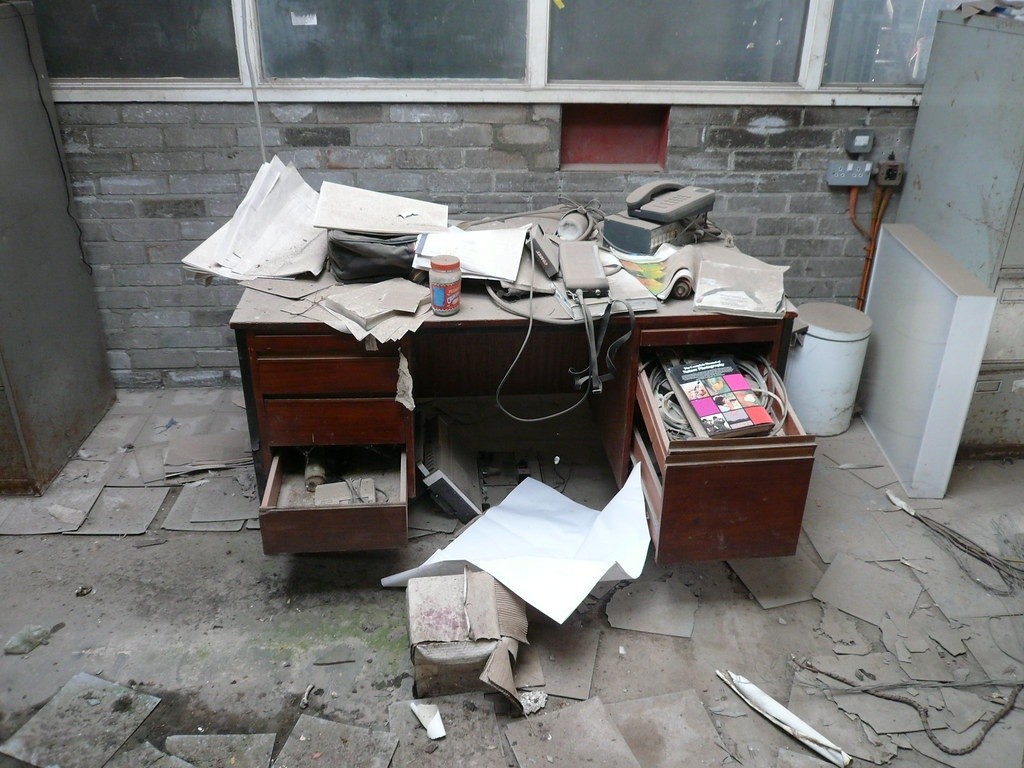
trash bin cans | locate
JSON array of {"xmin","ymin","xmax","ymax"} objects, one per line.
[{"xmin": 783, "ymin": 300, "xmax": 873, "ymax": 438}]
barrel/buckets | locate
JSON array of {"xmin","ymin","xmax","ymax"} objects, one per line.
[{"xmin": 783, "ymin": 302, "xmax": 872, "ymax": 437}]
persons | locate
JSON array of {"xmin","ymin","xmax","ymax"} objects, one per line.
[{"xmin": 689, "ymin": 379, "xmax": 735, "ymax": 433}]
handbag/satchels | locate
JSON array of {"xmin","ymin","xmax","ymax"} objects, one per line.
[{"xmin": 327, "ymin": 228, "xmax": 417, "ymax": 285}]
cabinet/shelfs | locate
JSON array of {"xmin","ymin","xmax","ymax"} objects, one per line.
[{"xmin": 230, "ymin": 219, "xmax": 818, "ymax": 566}]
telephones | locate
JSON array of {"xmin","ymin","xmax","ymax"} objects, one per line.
[{"xmin": 626, "ymin": 180, "xmax": 716, "ymax": 223}]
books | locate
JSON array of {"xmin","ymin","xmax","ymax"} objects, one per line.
[
  {"xmin": 670, "ymin": 356, "xmax": 776, "ymax": 439},
  {"xmin": 693, "ymin": 260, "xmax": 787, "ymax": 320},
  {"xmin": 326, "ymin": 278, "xmax": 432, "ymax": 330},
  {"xmin": 551, "ymin": 261, "xmax": 659, "ymax": 321}
]
